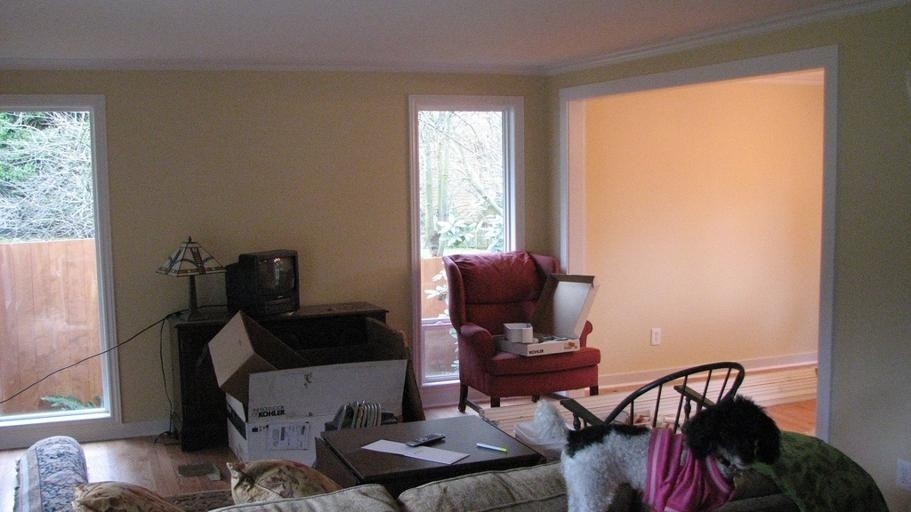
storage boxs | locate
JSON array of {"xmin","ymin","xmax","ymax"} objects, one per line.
[
  {"xmin": 224, "ymin": 357, "xmax": 408, "ymax": 468},
  {"xmin": 207, "ymin": 312, "xmax": 312, "ymax": 407}
]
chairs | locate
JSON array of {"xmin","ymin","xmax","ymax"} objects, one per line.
[
  {"xmin": 560, "ymin": 362, "xmax": 745, "ymax": 433},
  {"xmin": 442, "ymin": 249, "xmax": 601, "ymax": 412}
]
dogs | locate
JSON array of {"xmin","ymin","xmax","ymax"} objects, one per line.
[{"xmin": 532, "ymin": 393, "xmax": 782, "ymax": 511}]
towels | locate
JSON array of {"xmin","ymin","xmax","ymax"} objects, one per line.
[{"xmin": 752, "ymin": 432, "xmax": 888, "ymax": 512}]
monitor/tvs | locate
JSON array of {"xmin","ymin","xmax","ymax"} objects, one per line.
[{"xmin": 225, "ymin": 249, "xmax": 300, "ymax": 317}]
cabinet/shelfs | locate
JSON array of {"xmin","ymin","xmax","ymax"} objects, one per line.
[{"xmin": 165, "ymin": 302, "xmax": 389, "ymax": 452}]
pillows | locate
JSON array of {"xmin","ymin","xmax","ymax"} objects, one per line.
[
  {"xmin": 464, "ymin": 300, "xmax": 537, "ymax": 335},
  {"xmin": 72, "ymin": 481, "xmax": 187, "ymax": 512},
  {"xmin": 453, "ymin": 250, "xmax": 546, "ymax": 304},
  {"xmin": 226, "ymin": 459, "xmax": 343, "ymax": 505}
]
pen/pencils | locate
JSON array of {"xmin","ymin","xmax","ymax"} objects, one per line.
[{"xmin": 476, "ymin": 442, "xmax": 510, "ymax": 453}]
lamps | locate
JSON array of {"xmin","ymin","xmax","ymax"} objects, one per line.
[{"xmin": 156, "ymin": 236, "xmax": 228, "ymax": 321}]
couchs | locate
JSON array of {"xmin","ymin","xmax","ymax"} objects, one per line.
[{"xmin": 10, "ymin": 436, "xmax": 888, "ymax": 512}]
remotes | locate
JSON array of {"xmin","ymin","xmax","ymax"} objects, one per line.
[{"xmin": 406, "ymin": 433, "xmax": 446, "ymax": 447}]
